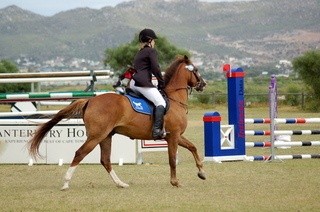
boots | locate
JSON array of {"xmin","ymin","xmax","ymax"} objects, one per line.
[{"xmin": 152, "ymin": 105, "xmax": 166, "ymax": 140}]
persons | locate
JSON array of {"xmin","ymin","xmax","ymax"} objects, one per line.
[{"xmin": 124, "ymin": 28, "xmax": 168, "ymax": 140}]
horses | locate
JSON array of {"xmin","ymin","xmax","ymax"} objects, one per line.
[{"xmin": 27, "ymin": 55, "xmax": 207, "ymax": 192}]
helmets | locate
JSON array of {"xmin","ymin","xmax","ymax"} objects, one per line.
[{"xmin": 139, "ymin": 29, "xmax": 159, "ymax": 42}]
